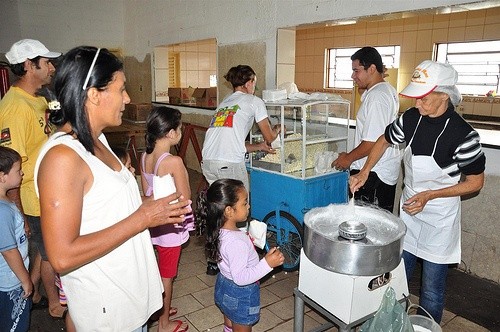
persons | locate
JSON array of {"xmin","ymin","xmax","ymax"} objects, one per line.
[
  {"xmin": 33, "ymin": 45, "xmax": 194, "ymax": 332},
  {"xmin": 329, "ymin": 45, "xmax": 401, "ymax": 289},
  {"xmin": 194, "ymin": 178, "xmax": 285, "ymax": 332},
  {"xmin": 0, "ymin": 39, "xmax": 69, "ymax": 319},
  {"xmin": 348, "ymin": 59, "xmax": 487, "ymax": 325},
  {"xmin": 0, "ymin": 145, "xmax": 33, "ymax": 332},
  {"xmin": 200, "ymin": 64, "xmax": 287, "ymax": 275},
  {"xmin": 140, "ymin": 106, "xmax": 196, "ymax": 332},
  {"xmin": 112, "ymin": 146, "xmax": 136, "ymax": 177}
]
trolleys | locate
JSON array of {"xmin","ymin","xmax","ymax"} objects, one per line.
[{"xmin": 245, "ymin": 96, "xmax": 350, "ymax": 273}]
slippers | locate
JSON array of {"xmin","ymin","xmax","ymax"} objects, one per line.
[
  {"xmin": 169, "ymin": 308, "xmax": 177, "ymax": 317},
  {"xmin": 49, "ymin": 307, "xmax": 68, "ymax": 319},
  {"xmin": 32, "ymin": 296, "xmax": 48, "ymax": 309},
  {"xmin": 172, "ymin": 320, "xmax": 189, "ymax": 332}
]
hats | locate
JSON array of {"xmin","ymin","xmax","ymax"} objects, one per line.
[
  {"xmin": 398, "ymin": 60, "xmax": 458, "ymax": 98},
  {"xmin": 5, "ymin": 39, "xmax": 61, "ymax": 64}
]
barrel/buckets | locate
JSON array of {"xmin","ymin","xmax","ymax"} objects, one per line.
[{"xmin": 407, "ymin": 304, "xmax": 442, "ymax": 332}]
